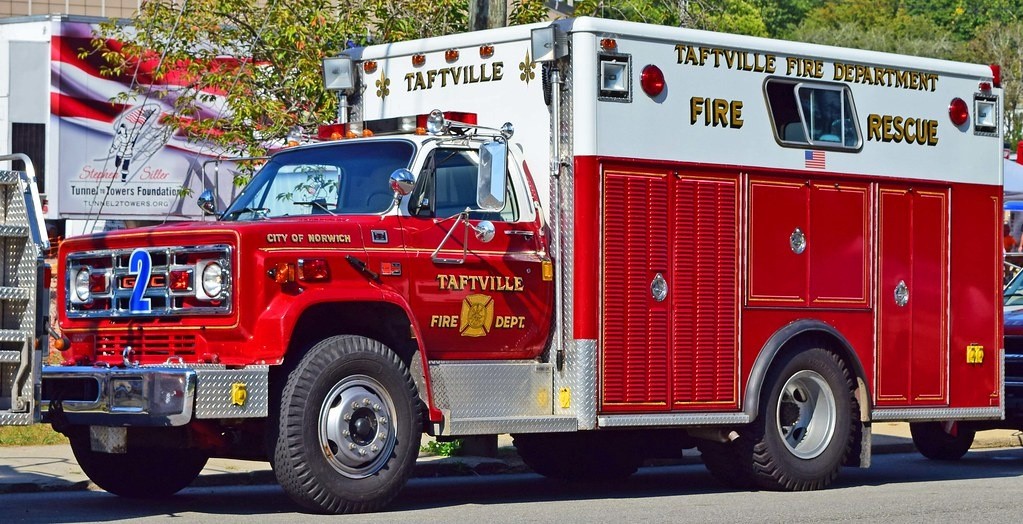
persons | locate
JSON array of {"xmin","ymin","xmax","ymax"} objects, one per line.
[{"xmin": 1002, "ymin": 224, "xmax": 1023, "ymax": 253}]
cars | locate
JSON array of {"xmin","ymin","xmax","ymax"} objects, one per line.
[{"xmin": 906, "ymin": 266, "xmax": 1023, "ymax": 460}]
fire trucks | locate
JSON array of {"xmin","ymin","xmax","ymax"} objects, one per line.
[{"xmin": 0, "ymin": 13, "xmax": 1003, "ymax": 509}]
